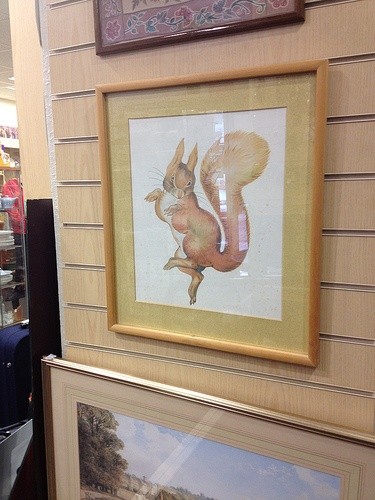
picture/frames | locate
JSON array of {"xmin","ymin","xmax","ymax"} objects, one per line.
[
  {"xmin": 40, "ymin": 355, "xmax": 375, "ymax": 499},
  {"xmin": 92, "ymin": 57, "xmax": 331, "ymax": 372},
  {"xmin": 91, "ymin": 0, "xmax": 306, "ymax": 57}
]
